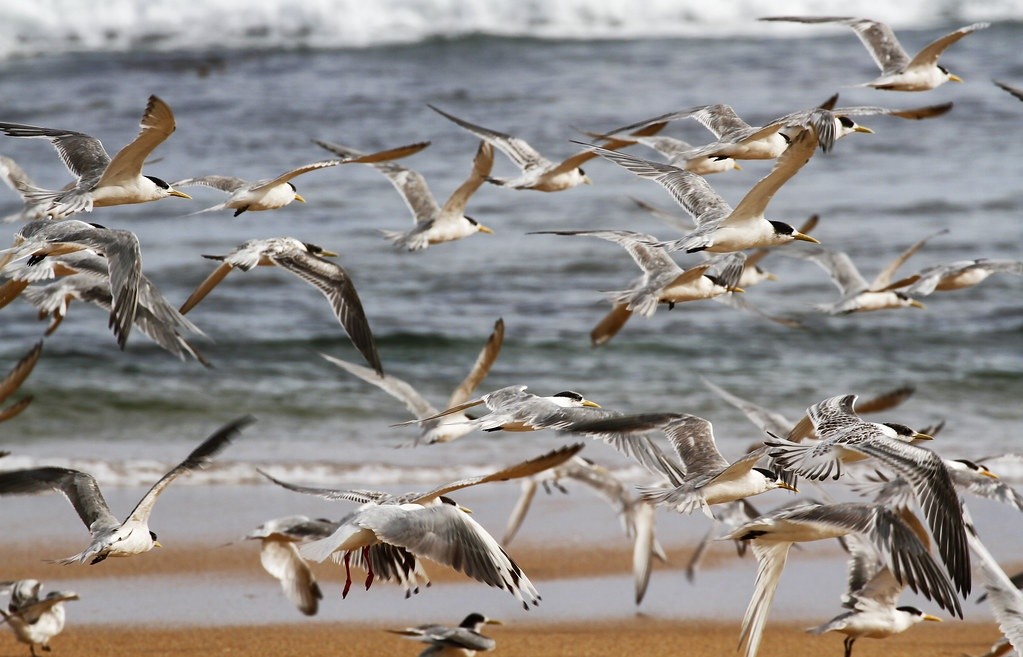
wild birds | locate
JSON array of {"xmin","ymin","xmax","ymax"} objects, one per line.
[
  {"xmin": 0, "ymin": 83, "xmax": 956, "ymax": 378},
  {"xmin": 244, "ymin": 315, "xmax": 1023, "ymax": 657},
  {"xmin": 990, "ymin": 78, "xmax": 1023, "ymax": 102},
  {"xmin": 754, "ymin": 14, "xmax": 993, "ymax": 92},
  {"xmin": 0, "ymin": 579, "xmax": 82, "ymax": 656},
  {"xmin": 788, "ymin": 228, "xmax": 1022, "ymax": 312},
  {"xmin": 0, "ymin": 334, "xmax": 260, "ymax": 565}
]
